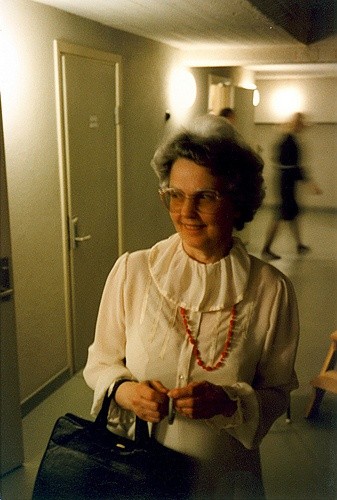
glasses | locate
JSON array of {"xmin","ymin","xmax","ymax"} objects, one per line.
[{"xmin": 158, "ymin": 178, "xmax": 230, "ymax": 215}]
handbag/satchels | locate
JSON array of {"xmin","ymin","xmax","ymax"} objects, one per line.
[{"xmin": 31, "ymin": 379, "xmax": 201, "ymax": 500}]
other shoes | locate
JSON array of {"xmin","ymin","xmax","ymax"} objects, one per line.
[
  {"xmin": 297, "ymin": 245, "xmax": 310, "ymax": 254},
  {"xmin": 262, "ymin": 250, "xmax": 280, "ymax": 260}
]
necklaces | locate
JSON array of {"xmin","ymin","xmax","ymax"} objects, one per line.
[{"xmin": 180, "ymin": 307, "xmax": 236, "ymax": 371}]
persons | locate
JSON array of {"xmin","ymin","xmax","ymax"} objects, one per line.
[
  {"xmin": 81, "ymin": 116, "xmax": 299, "ymax": 500},
  {"xmin": 261, "ymin": 113, "xmax": 321, "ymax": 262}
]
